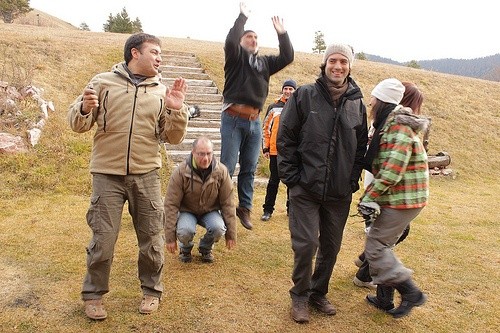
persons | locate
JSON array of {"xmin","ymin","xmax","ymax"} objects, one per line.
[
  {"xmin": 67, "ymin": 33, "xmax": 188, "ymax": 320},
  {"xmin": 276, "ymin": 43, "xmax": 369, "ymax": 324},
  {"xmin": 259, "ymin": 80, "xmax": 296, "ymax": 220},
  {"xmin": 351, "ymin": 78, "xmax": 431, "ymax": 319},
  {"xmin": 163, "ymin": 136, "xmax": 238, "ymax": 263},
  {"xmin": 220, "ymin": 1, "xmax": 294, "ymax": 230}
]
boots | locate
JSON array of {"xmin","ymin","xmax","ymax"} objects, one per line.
[
  {"xmin": 392, "ymin": 278, "xmax": 428, "ymax": 318},
  {"xmin": 366, "ymin": 283, "xmax": 395, "ymax": 311}
]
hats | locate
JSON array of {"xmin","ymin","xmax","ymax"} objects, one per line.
[
  {"xmin": 323, "ymin": 41, "xmax": 354, "ymax": 69},
  {"xmin": 281, "ymin": 79, "xmax": 296, "ymax": 92},
  {"xmin": 370, "ymin": 78, "xmax": 405, "ymax": 105}
]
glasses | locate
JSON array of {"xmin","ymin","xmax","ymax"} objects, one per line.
[{"xmin": 193, "ymin": 151, "xmax": 212, "ymax": 157}]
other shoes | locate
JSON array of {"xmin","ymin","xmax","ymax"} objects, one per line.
[
  {"xmin": 355, "ymin": 256, "xmax": 364, "ymax": 267},
  {"xmin": 261, "ymin": 211, "xmax": 272, "ymax": 221},
  {"xmin": 291, "ymin": 299, "xmax": 310, "ymax": 323},
  {"xmin": 139, "ymin": 294, "xmax": 159, "ymax": 314},
  {"xmin": 353, "ymin": 276, "xmax": 377, "ymax": 290},
  {"xmin": 83, "ymin": 299, "xmax": 107, "ymax": 320},
  {"xmin": 236, "ymin": 206, "xmax": 253, "ymax": 230},
  {"xmin": 180, "ymin": 246, "xmax": 193, "ymax": 262},
  {"xmin": 200, "ymin": 247, "xmax": 214, "ymax": 262},
  {"xmin": 309, "ymin": 297, "xmax": 336, "ymax": 315}
]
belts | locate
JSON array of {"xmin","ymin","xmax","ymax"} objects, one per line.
[{"xmin": 224, "ymin": 109, "xmax": 259, "ymax": 121}]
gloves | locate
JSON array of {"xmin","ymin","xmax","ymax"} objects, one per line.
[{"xmin": 358, "ymin": 199, "xmax": 381, "ymax": 216}]
